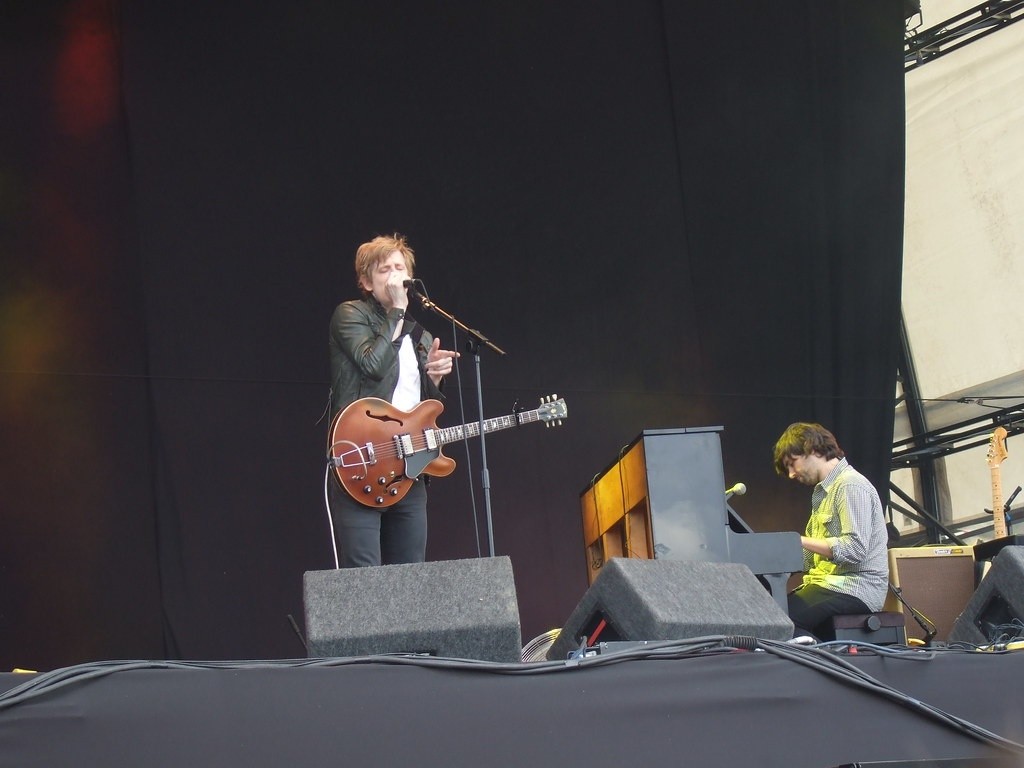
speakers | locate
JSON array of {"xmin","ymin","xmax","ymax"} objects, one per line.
[
  {"xmin": 545, "ymin": 557, "xmax": 795, "ymax": 661},
  {"xmin": 949, "ymin": 545, "xmax": 1024, "ymax": 648},
  {"xmin": 302, "ymin": 556, "xmax": 523, "ymax": 664}
]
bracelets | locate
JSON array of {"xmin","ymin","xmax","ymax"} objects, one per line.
[{"xmin": 401, "ymin": 314, "xmax": 405, "ymax": 319}]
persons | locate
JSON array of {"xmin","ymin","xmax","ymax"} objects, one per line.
[
  {"xmin": 774, "ymin": 422, "xmax": 889, "ymax": 632},
  {"xmin": 328, "ymin": 234, "xmax": 460, "ymax": 569}
]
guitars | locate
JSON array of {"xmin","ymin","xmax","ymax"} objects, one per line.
[{"xmin": 325, "ymin": 393, "xmax": 568, "ymax": 509}]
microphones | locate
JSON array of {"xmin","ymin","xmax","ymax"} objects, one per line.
[
  {"xmin": 725, "ymin": 482, "xmax": 746, "ymax": 495},
  {"xmin": 403, "ymin": 279, "xmax": 421, "ymax": 287}
]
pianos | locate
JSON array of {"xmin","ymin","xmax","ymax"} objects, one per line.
[{"xmin": 580, "ymin": 425, "xmax": 806, "ymax": 620}]
